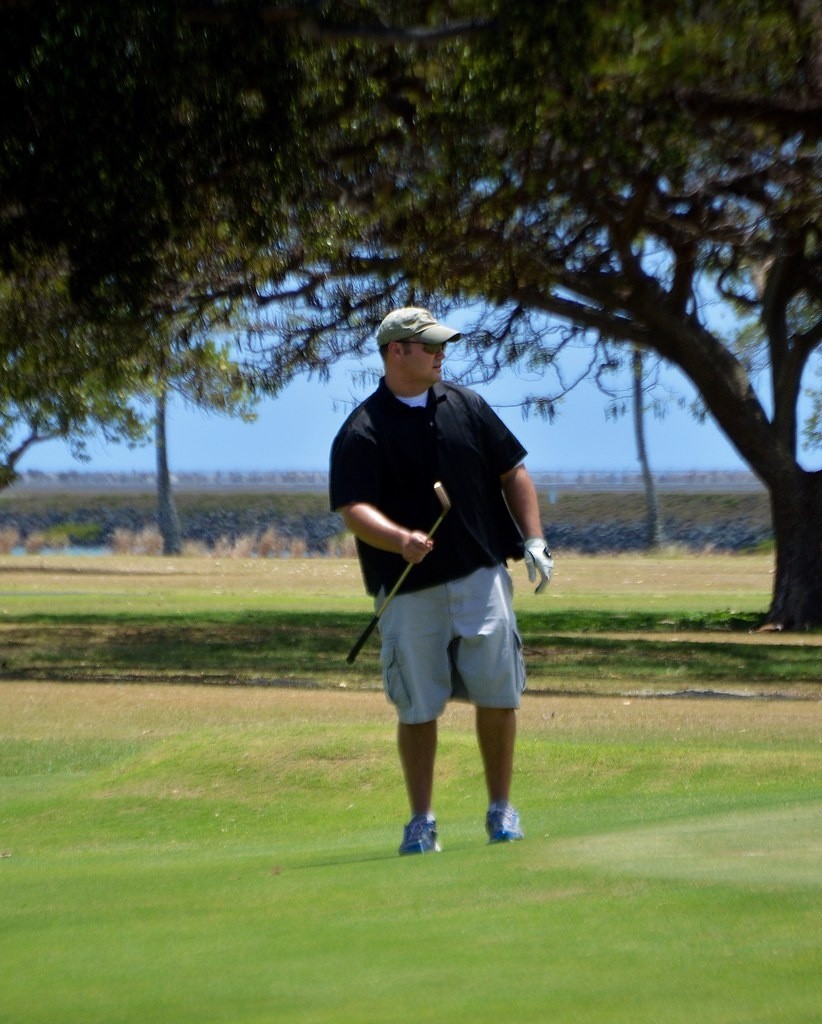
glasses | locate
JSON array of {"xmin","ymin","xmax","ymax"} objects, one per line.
[{"xmin": 395, "ymin": 338, "xmax": 446, "ymax": 355}]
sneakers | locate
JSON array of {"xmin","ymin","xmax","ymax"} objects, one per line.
[
  {"xmin": 485, "ymin": 802, "xmax": 524, "ymax": 845},
  {"xmin": 399, "ymin": 814, "xmax": 440, "ymax": 854}
]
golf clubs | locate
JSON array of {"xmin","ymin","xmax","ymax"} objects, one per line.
[{"xmin": 345, "ymin": 480, "xmax": 452, "ymax": 666}]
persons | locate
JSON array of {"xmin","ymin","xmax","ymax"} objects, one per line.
[{"xmin": 330, "ymin": 307, "xmax": 553, "ymax": 856}]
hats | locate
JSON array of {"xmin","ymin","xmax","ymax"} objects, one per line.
[{"xmin": 377, "ymin": 308, "xmax": 460, "ymax": 346}]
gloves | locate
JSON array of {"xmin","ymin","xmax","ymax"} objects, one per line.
[{"xmin": 524, "ymin": 538, "xmax": 553, "ymax": 594}]
